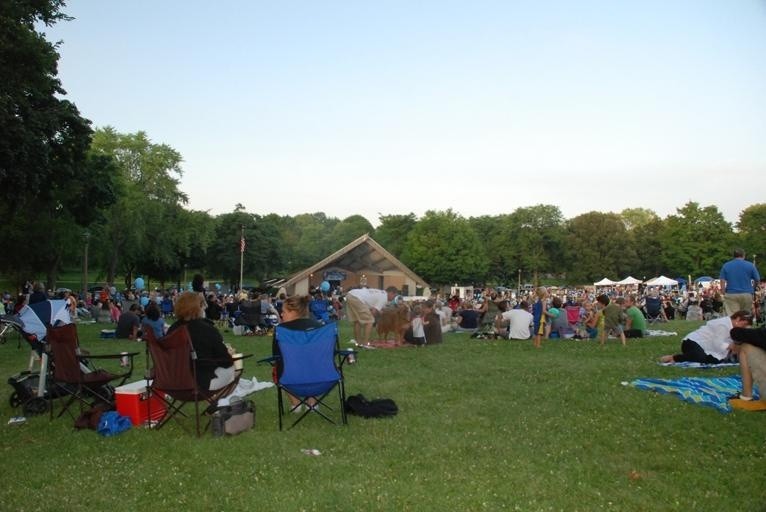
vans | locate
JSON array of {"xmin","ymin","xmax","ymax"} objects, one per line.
[{"xmin": 525, "ymin": 284, "xmax": 534, "ymax": 291}]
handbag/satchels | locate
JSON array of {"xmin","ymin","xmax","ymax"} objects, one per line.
[
  {"xmin": 345, "ymin": 394, "xmax": 398, "ymax": 418},
  {"xmin": 212, "ymin": 395, "xmax": 256, "ymax": 435}
]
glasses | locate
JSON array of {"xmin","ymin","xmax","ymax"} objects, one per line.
[{"xmin": 744, "ymin": 312, "xmax": 753, "ymax": 319}]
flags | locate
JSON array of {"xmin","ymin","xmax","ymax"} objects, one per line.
[{"xmin": 240, "ymin": 236, "xmax": 245, "ymax": 254}]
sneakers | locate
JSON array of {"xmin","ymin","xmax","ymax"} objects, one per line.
[
  {"xmin": 290, "ymin": 406, "xmax": 302, "ymax": 412},
  {"xmin": 571, "ymin": 334, "xmax": 577, "ymax": 340},
  {"xmin": 355, "ymin": 341, "xmax": 370, "ymax": 347},
  {"xmin": 304, "ymin": 404, "xmax": 319, "ymax": 413},
  {"xmin": 359, "ymin": 343, "xmax": 375, "ymax": 350},
  {"xmin": 727, "ymin": 390, "xmax": 754, "ymax": 401},
  {"xmin": 575, "ymin": 335, "xmax": 587, "ymax": 341}
]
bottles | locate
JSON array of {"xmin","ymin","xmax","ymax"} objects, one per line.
[{"xmin": 347, "ymin": 348, "xmax": 356, "ymax": 364}]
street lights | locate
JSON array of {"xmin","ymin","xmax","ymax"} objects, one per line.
[
  {"xmin": 752, "ymin": 254, "xmax": 758, "ymax": 265},
  {"xmin": 82, "ymin": 227, "xmax": 92, "ymax": 291}
]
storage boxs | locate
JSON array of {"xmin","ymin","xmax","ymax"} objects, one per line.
[{"xmin": 115, "ymin": 379, "xmax": 168, "ymax": 426}]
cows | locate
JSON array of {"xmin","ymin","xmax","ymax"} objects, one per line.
[{"xmin": 373, "ymin": 302, "xmax": 411, "ymax": 347}]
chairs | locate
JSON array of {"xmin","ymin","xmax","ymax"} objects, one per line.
[
  {"xmin": 140, "ymin": 325, "xmax": 253, "ymax": 436},
  {"xmin": 141, "ymin": 324, "xmax": 155, "ymax": 369},
  {"xmin": 240, "ymin": 301, "xmax": 274, "ymax": 336},
  {"xmin": 47, "ymin": 323, "xmax": 139, "ymax": 421},
  {"xmin": 273, "ymin": 322, "xmax": 348, "ymax": 431},
  {"xmin": 309, "ymin": 300, "xmax": 336, "ymax": 319},
  {"xmin": 646, "ymin": 296, "xmax": 663, "ymax": 325},
  {"xmin": 160, "ymin": 301, "xmax": 172, "ymax": 318}
]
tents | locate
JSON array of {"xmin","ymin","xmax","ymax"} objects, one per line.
[{"xmin": 594, "ymin": 275, "xmax": 678, "ymax": 293}]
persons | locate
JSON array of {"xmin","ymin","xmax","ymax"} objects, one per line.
[
  {"xmin": 345, "ymin": 287, "xmax": 397, "ymax": 349},
  {"xmin": 166, "ymin": 292, "xmax": 235, "ymax": 417},
  {"xmin": 497, "ymin": 282, "xmax": 723, "ymax": 348},
  {"xmin": 272, "ymin": 296, "xmax": 324, "ymax": 413},
  {"xmin": 661, "ymin": 311, "xmax": 753, "ymax": 363},
  {"xmin": 192, "ymin": 273, "xmax": 343, "ymax": 333},
  {"xmin": 720, "ymin": 248, "xmax": 761, "ymax": 315},
  {"xmin": 389, "ymin": 287, "xmax": 502, "ymax": 349},
  {"xmin": 730, "ymin": 323, "xmax": 766, "ymax": 402},
  {"xmin": 1, "ymin": 272, "xmax": 177, "ymax": 342}
]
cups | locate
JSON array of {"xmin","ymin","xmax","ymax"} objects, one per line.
[
  {"xmin": 232, "ymin": 352, "xmax": 244, "ymax": 370},
  {"xmin": 120, "ymin": 352, "xmax": 129, "ymax": 367}
]
